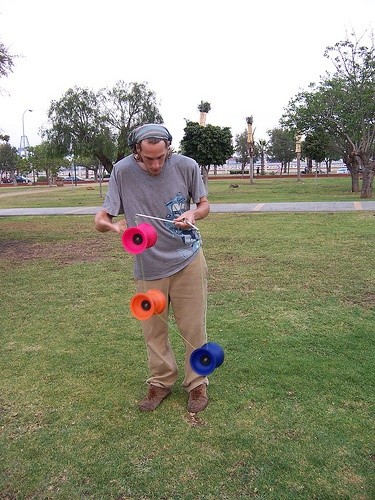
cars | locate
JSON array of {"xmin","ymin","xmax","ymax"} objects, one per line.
[
  {"xmin": 337, "ymin": 168, "xmax": 350, "ymax": 174},
  {"xmin": 3, "ymin": 176, "xmax": 32, "ymax": 183},
  {"xmin": 63, "ymin": 177, "xmax": 85, "ymax": 181}
]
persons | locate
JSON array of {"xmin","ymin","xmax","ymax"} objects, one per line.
[
  {"xmin": 95, "ymin": 124, "xmax": 211, "ymax": 412},
  {"xmin": 256, "ymin": 167, "xmax": 259, "ymax": 174},
  {"xmin": 316, "ymin": 166, "xmax": 320, "ymax": 173},
  {"xmin": 301, "ymin": 167, "xmax": 310, "ymax": 174},
  {"xmin": 214, "ymin": 169, "xmax": 217, "ymax": 175},
  {"xmin": 69, "ymin": 172, "xmax": 71, "ymax": 177}
]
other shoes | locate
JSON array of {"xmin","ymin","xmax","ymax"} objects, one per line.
[
  {"xmin": 138, "ymin": 385, "xmax": 171, "ymax": 413},
  {"xmin": 188, "ymin": 383, "xmax": 208, "ymax": 412}
]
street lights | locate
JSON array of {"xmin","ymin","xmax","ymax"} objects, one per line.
[{"xmin": 22, "ymin": 109, "xmax": 32, "ymax": 161}]
